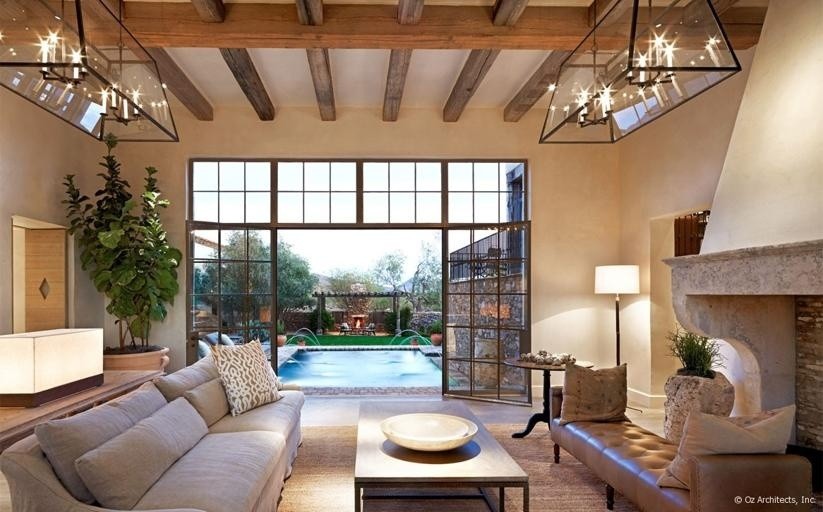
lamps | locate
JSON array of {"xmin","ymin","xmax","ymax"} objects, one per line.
[
  {"xmin": 1, "ymin": 1, "xmax": 180, "ymax": 143},
  {"xmin": 594, "ymin": 264, "xmax": 643, "ymax": 415},
  {"xmin": 537, "ymin": 0, "xmax": 743, "ymax": 144}
]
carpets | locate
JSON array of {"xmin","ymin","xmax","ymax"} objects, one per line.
[{"xmin": 277, "ymin": 423, "xmax": 644, "ymax": 512}]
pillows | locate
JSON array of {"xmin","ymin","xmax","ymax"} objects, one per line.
[
  {"xmin": 182, "ymin": 375, "xmax": 230, "ymax": 423},
  {"xmin": 560, "ymin": 365, "xmax": 628, "ymax": 425},
  {"xmin": 656, "ymin": 404, "xmax": 797, "ymax": 487},
  {"xmin": 76, "ymin": 398, "xmax": 211, "ymax": 509},
  {"xmin": 155, "ymin": 356, "xmax": 220, "ymax": 403},
  {"xmin": 210, "ymin": 339, "xmax": 283, "ymax": 416},
  {"xmin": 34, "ymin": 379, "xmax": 168, "ymax": 505}
]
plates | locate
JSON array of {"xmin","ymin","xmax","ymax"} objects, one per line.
[{"xmin": 379, "ymin": 412, "xmax": 478, "ymax": 451}]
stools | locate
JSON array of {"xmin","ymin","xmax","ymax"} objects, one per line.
[{"xmin": 341, "ymin": 327, "xmax": 377, "ymax": 335}]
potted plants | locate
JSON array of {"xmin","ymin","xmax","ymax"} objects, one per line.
[
  {"xmin": 662, "ymin": 325, "xmax": 735, "ymax": 439},
  {"xmin": 63, "ymin": 133, "xmax": 182, "ymax": 375}
]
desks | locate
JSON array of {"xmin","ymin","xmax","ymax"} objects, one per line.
[{"xmin": 0, "ymin": 369, "xmax": 166, "ymax": 446}]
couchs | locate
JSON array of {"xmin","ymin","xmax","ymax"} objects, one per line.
[
  {"xmin": 547, "ymin": 379, "xmax": 813, "ymax": 511},
  {"xmin": 0, "ymin": 383, "xmax": 307, "ymax": 511}
]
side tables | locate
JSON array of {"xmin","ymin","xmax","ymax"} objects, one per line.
[{"xmin": 503, "ymin": 353, "xmax": 596, "ymax": 438}]
